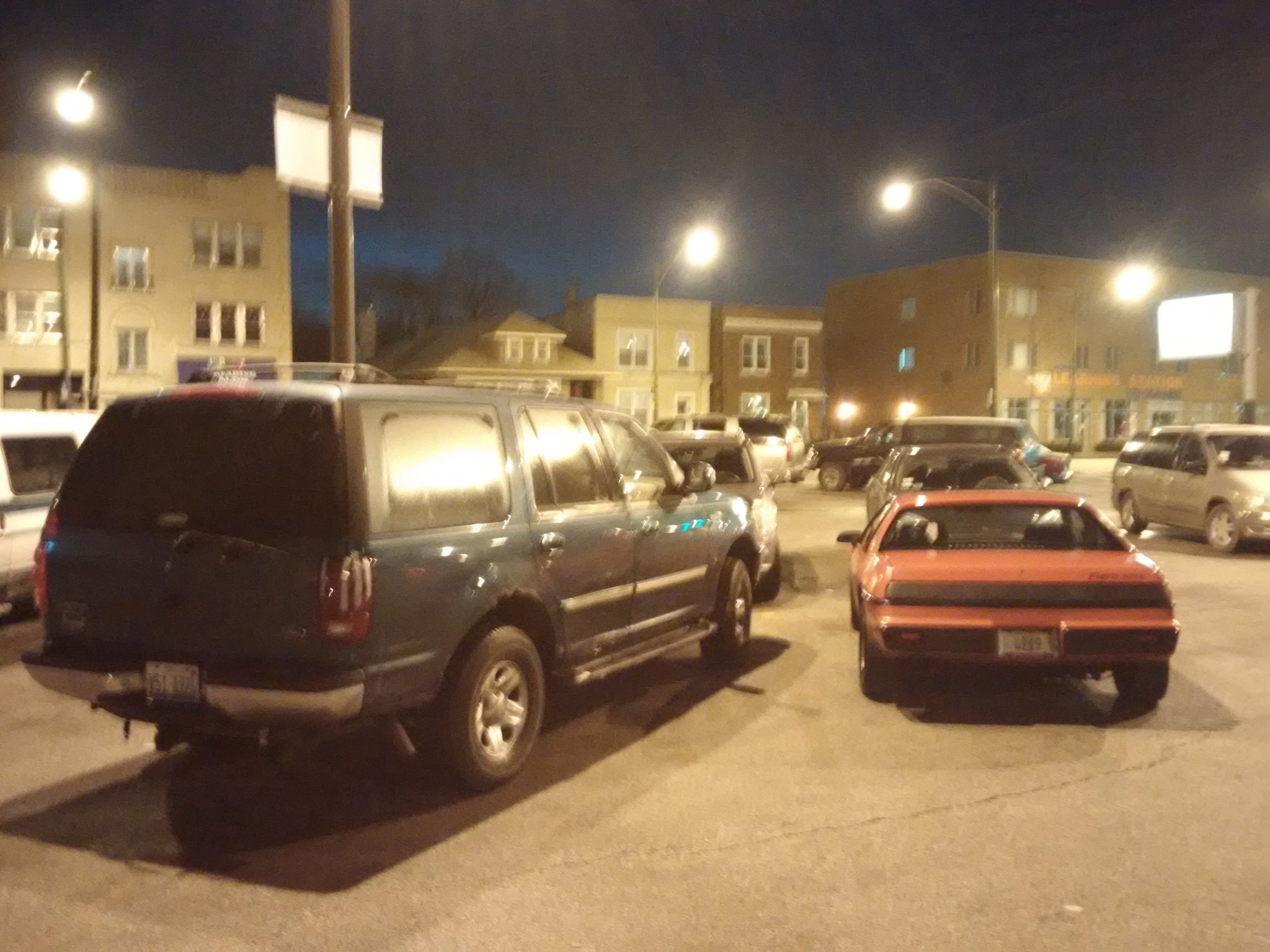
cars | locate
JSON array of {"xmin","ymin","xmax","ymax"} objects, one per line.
[
  {"xmin": 1, "ymin": 404, "xmax": 103, "ymax": 621},
  {"xmin": 835, "ymin": 485, "xmax": 1181, "ymax": 706},
  {"xmin": 734, "ymin": 409, "xmax": 1076, "ymax": 490},
  {"xmin": 863, "ymin": 441, "xmax": 1053, "ymax": 535}
]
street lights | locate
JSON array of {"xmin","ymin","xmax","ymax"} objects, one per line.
[
  {"xmin": 52, "ymin": 68, "xmax": 107, "ymax": 410},
  {"xmin": 1103, "ymin": 261, "xmax": 1154, "ymax": 438},
  {"xmin": 880, "ymin": 168, "xmax": 1004, "ymax": 418},
  {"xmin": 47, "ymin": 150, "xmax": 90, "ymax": 408},
  {"xmin": 649, "ymin": 219, "xmax": 723, "ymax": 424}
]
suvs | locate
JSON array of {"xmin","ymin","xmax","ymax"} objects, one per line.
[
  {"xmin": 646, "ymin": 413, "xmax": 782, "ymax": 600},
  {"xmin": 18, "ymin": 364, "xmax": 764, "ymax": 791},
  {"xmin": 1109, "ymin": 421, "xmax": 1270, "ymax": 555}
]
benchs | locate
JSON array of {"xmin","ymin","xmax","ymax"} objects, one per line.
[{"xmin": 910, "ymin": 458, "xmax": 1022, "ymax": 488}]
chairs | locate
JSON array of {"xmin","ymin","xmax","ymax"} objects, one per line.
[
  {"xmin": 892, "ymin": 520, "xmax": 949, "ymax": 550},
  {"xmin": 1021, "ymin": 523, "xmax": 1072, "ymax": 549}
]
